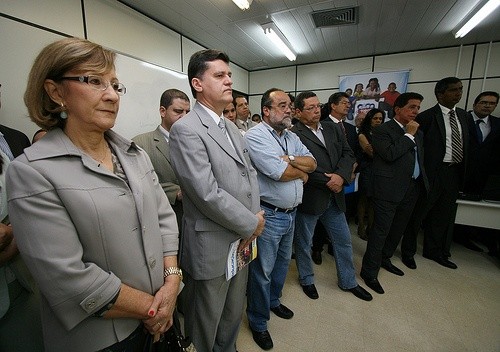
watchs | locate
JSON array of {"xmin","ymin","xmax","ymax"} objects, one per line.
[
  {"xmin": 163, "ymin": 266, "xmax": 183, "ymax": 281},
  {"xmin": 288, "ymin": 154, "xmax": 296, "ymax": 165}
]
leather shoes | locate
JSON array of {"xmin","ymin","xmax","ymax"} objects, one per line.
[
  {"xmin": 360, "ymin": 272, "xmax": 384, "ymax": 294},
  {"xmin": 248, "ymin": 324, "xmax": 273, "ymax": 351},
  {"xmin": 311, "ymin": 249, "xmax": 322, "ymax": 265},
  {"xmin": 401, "ymin": 256, "xmax": 416, "ymax": 269},
  {"xmin": 328, "ymin": 244, "xmax": 334, "ymax": 256},
  {"xmin": 270, "ymin": 304, "xmax": 294, "ymax": 319},
  {"xmin": 427, "ymin": 257, "xmax": 458, "ymax": 269},
  {"xmin": 338, "ymin": 285, "xmax": 373, "ymax": 301},
  {"xmin": 381, "ymin": 259, "xmax": 404, "ymax": 276},
  {"xmin": 301, "ymin": 284, "xmax": 318, "ymax": 299}
]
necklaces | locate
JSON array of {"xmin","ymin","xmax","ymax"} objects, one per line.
[
  {"xmin": 268, "ymin": 128, "xmax": 288, "ymax": 156},
  {"xmin": 70, "ymin": 139, "xmax": 108, "ymax": 164}
]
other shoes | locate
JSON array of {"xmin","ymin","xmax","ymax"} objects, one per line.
[{"xmin": 358, "ymin": 225, "xmax": 368, "ymax": 240}]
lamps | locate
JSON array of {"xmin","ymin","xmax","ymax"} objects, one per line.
[
  {"xmin": 232, "ymin": 0, "xmax": 254, "ymax": 12},
  {"xmin": 450, "ymin": 0, "xmax": 500, "ymax": 40},
  {"xmin": 260, "ymin": 21, "xmax": 298, "ymax": 61}
]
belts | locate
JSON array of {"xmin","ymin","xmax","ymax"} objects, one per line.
[{"xmin": 260, "ymin": 199, "xmax": 296, "ymax": 214}]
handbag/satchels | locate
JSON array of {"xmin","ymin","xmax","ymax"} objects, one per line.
[{"xmin": 151, "ymin": 310, "xmax": 198, "ymax": 352}]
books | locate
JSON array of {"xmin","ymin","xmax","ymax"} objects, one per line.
[{"xmin": 225, "ymin": 235, "xmax": 257, "ymax": 282}]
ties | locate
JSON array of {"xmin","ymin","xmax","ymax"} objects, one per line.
[
  {"xmin": 476, "ymin": 119, "xmax": 483, "ymax": 144},
  {"xmin": 338, "ymin": 122, "xmax": 347, "ymax": 138},
  {"xmin": 218, "ymin": 118, "xmax": 228, "ymax": 141},
  {"xmin": 244, "ymin": 122, "xmax": 248, "ymax": 131},
  {"xmin": 448, "ymin": 109, "xmax": 463, "ymax": 163},
  {"xmin": 413, "ymin": 146, "xmax": 420, "ymax": 179}
]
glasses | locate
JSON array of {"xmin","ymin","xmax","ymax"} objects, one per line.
[
  {"xmin": 269, "ymin": 104, "xmax": 293, "ymax": 112},
  {"xmin": 302, "ymin": 103, "xmax": 324, "ymax": 110},
  {"xmin": 54, "ymin": 75, "xmax": 126, "ymax": 96}
]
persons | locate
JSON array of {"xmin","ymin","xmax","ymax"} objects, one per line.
[
  {"xmin": 130, "ymin": 89, "xmax": 191, "ymax": 347},
  {"xmin": 467, "ymin": 91, "xmax": 500, "ymax": 202},
  {"xmin": 243, "ymin": 88, "xmax": 317, "ymax": 351},
  {"xmin": 342, "ymin": 77, "xmax": 401, "ymax": 120},
  {"xmin": 4, "ymin": 36, "xmax": 183, "ymax": 352},
  {"xmin": 400, "ymin": 77, "xmax": 470, "ymax": 270},
  {"xmin": 360, "ymin": 92, "xmax": 431, "ymax": 295},
  {"xmin": 0, "ymin": 84, "xmax": 48, "ymax": 352},
  {"xmin": 289, "ymin": 90, "xmax": 373, "ymax": 302},
  {"xmin": 169, "ymin": 50, "xmax": 265, "ymax": 352},
  {"xmin": 222, "ymin": 92, "xmax": 389, "ymax": 265},
  {"xmin": 32, "ymin": 129, "xmax": 47, "ymax": 144}
]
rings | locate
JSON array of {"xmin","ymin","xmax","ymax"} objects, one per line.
[{"xmin": 158, "ymin": 322, "xmax": 162, "ymax": 327}]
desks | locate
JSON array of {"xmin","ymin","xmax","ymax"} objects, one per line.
[{"xmin": 454, "ymin": 198, "xmax": 500, "ymax": 230}]
